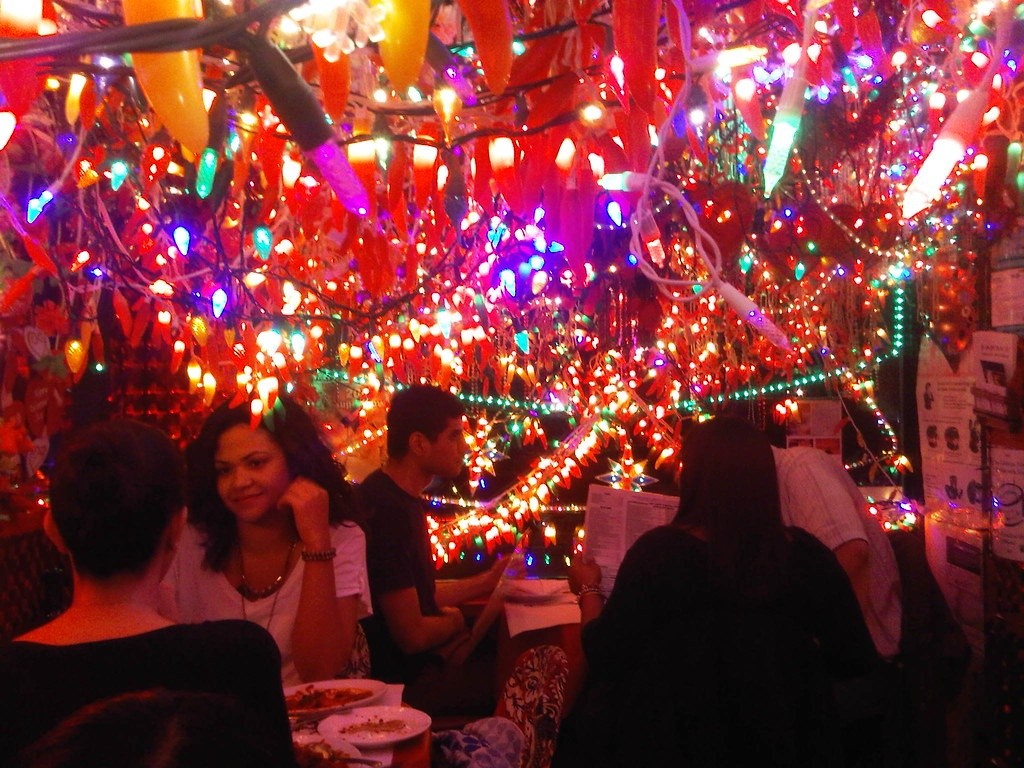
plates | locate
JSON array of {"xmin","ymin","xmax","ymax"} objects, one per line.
[
  {"xmin": 317, "ymin": 705, "xmax": 432, "ymax": 748},
  {"xmin": 500, "ymin": 577, "xmax": 566, "ymax": 604},
  {"xmin": 292, "ymin": 734, "xmax": 360, "ymax": 768},
  {"xmin": 283, "ymin": 679, "xmax": 388, "ymax": 718}
]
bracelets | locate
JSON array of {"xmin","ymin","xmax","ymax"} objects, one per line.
[
  {"xmin": 576, "ymin": 585, "xmax": 604, "ymax": 605},
  {"xmin": 302, "ymin": 549, "xmax": 337, "ymax": 560}
]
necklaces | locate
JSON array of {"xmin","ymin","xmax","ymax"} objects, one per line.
[{"xmin": 239, "ymin": 538, "xmax": 297, "ymax": 631}]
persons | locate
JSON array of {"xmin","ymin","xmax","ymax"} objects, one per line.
[
  {"xmin": 0, "ymin": 421, "xmax": 296, "ymax": 768},
  {"xmin": 355, "ymin": 387, "xmax": 524, "ymax": 713},
  {"xmin": 555, "ymin": 417, "xmax": 875, "ymax": 768},
  {"xmin": 774, "ymin": 444, "xmax": 911, "ymax": 768},
  {"xmin": 154, "ymin": 399, "xmax": 372, "ymax": 685},
  {"xmin": 966, "ymin": 419, "xmax": 981, "ymax": 454},
  {"xmin": 923, "ymin": 382, "xmax": 934, "ymax": 410}
]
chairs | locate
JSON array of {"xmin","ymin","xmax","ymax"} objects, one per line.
[{"xmin": 493, "ymin": 644, "xmax": 570, "ymax": 768}]
